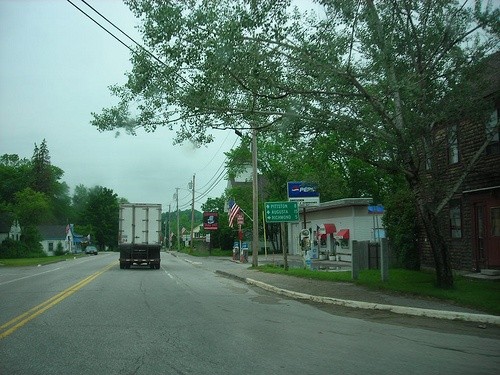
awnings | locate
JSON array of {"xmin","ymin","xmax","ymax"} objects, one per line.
[
  {"xmin": 319, "ymin": 224, "xmax": 336, "ymax": 234},
  {"xmin": 335, "ymin": 228, "xmax": 349, "ymax": 240}
]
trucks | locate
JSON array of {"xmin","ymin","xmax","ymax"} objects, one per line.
[{"xmin": 118, "ymin": 203, "xmax": 163, "ymax": 269}]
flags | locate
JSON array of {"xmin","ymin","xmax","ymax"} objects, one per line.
[
  {"xmin": 170, "ymin": 233, "xmax": 173, "ymax": 237},
  {"xmin": 228, "ymin": 200, "xmax": 240, "ymax": 228},
  {"xmin": 181, "ymin": 227, "xmax": 186, "ymax": 233}
]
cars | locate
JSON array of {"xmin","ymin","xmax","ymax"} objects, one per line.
[{"xmin": 85, "ymin": 245, "xmax": 98, "ymax": 255}]
